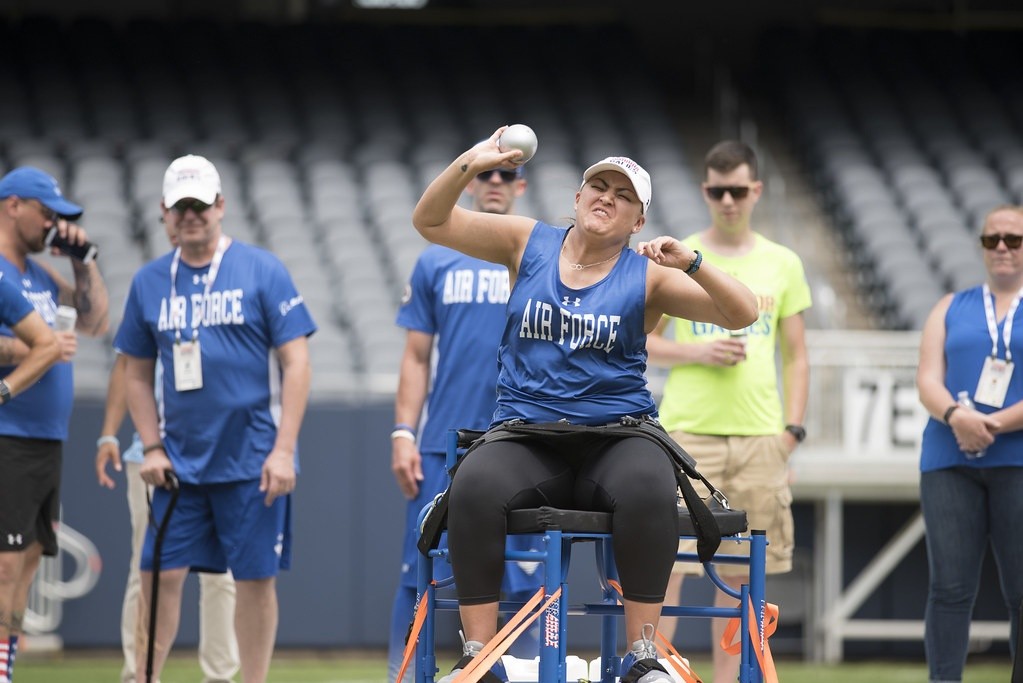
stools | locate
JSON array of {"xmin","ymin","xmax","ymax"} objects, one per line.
[{"xmin": 415, "ymin": 427, "xmax": 773, "ymax": 683}]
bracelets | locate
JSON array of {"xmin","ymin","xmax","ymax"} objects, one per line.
[
  {"xmin": 944, "ymin": 405, "xmax": 959, "ymax": 426},
  {"xmin": 390, "ymin": 430, "xmax": 415, "ymax": 443},
  {"xmin": 142, "ymin": 444, "xmax": 164, "ymax": 453},
  {"xmin": 97, "ymin": 435, "xmax": 120, "ymax": 447},
  {"xmin": 683, "ymin": 250, "xmax": 702, "ymax": 276},
  {"xmin": 390, "ymin": 424, "xmax": 416, "ymax": 436}
]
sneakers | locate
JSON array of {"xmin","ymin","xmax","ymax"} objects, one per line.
[
  {"xmin": 435, "ymin": 648, "xmax": 510, "ymax": 683},
  {"xmin": 619, "ymin": 639, "xmax": 675, "ymax": 683}
]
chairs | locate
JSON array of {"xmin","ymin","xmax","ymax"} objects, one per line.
[{"xmin": 0, "ymin": 83, "xmax": 1023, "ymax": 389}]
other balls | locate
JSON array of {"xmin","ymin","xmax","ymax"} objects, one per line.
[{"xmin": 499, "ymin": 123, "xmax": 538, "ymax": 164}]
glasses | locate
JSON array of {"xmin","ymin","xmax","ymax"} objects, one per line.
[
  {"xmin": 476, "ymin": 170, "xmax": 516, "ymax": 183},
  {"xmin": 705, "ymin": 185, "xmax": 750, "ymax": 199},
  {"xmin": 171, "ymin": 193, "xmax": 219, "ymax": 212},
  {"xmin": 22, "ymin": 201, "xmax": 60, "ymax": 223},
  {"xmin": 980, "ymin": 234, "xmax": 1023, "ymax": 250}
]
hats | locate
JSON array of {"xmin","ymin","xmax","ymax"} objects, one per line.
[
  {"xmin": 161, "ymin": 154, "xmax": 222, "ymax": 209},
  {"xmin": 579, "ymin": 156, "xmax": 653, "ymax": 217},
  {"xmin": 0, "ymin": 166, "xmax": 84, "ymax": 222},
  {"xmin": 473, "ymin": 137, "xmax": 526, "ymax": 178}
]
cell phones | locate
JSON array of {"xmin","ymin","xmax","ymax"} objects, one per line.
[{"xmin": 44, "ymin": 226, "xmax": 99, "ymax": 263}]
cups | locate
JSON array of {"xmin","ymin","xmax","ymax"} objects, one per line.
[{"xmin": 50, "ymin": 305, "xmax": 76, "ymax": 334}]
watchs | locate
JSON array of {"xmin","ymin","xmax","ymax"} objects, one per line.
[
  {"xmin": 786, "ymin": 426, "xmax": 806, "ymax": 443},
  {"xmin": 0, "ymin": 380, "xmax": 9, "ymax": 405}
]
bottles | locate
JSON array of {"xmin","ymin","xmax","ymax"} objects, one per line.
[{"xmin": 956, "ymin": 391, "xmax": 988, "ymax": 461}]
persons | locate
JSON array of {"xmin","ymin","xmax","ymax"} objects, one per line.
[
  {"xmin": 411, "ymin": 124, "xmax": 759, "ymax": 683},
  {"xmin": 916, "ymin": 207, "xmax": 1023, "ymax": 683},
  {"xmin": 112, "ymin": 153, "xmax": 319, "ymax": 683},
  {"xmin": 644, "ymin": 141, "xmax": 812, "ymax": 683},
  {"xmin": 0, "ymin": 272, "xmax": 60, "ymax": 407},
  {"xmin": 0, "ymin": 165, "xmax": 109, "ymax": 683},
  {"xmin": 388, "ymin": 164, "xmax": 545, "ymax": 683},
  {"xmin": 95, "ymin": 345, "xmax": 281, "ymax": 683}
]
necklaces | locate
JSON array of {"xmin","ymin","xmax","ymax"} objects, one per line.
[{"xmin": 561, "ymin": 227, "xmax": 622, "ymax": 269}]
